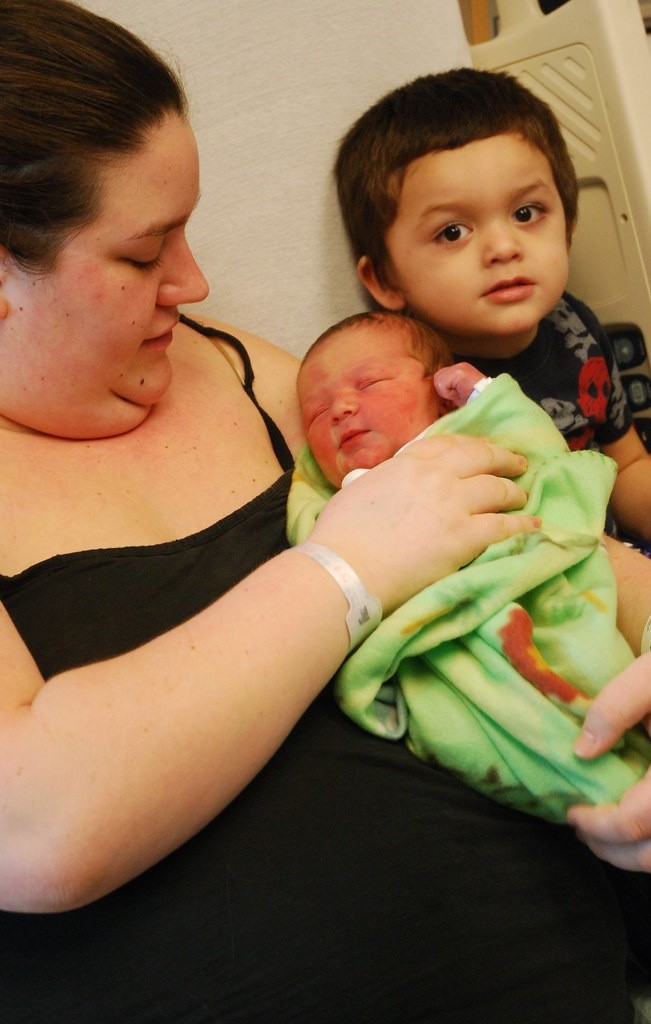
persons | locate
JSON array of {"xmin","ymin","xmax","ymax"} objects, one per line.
[{"xmin": 0, "ymin": 0, "xmax": 651, "ymax": 1024}]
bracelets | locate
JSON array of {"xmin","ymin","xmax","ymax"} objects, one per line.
[{"xmin": 290, "ymin": 541, "xmax": 382, "ymax": 654}]
